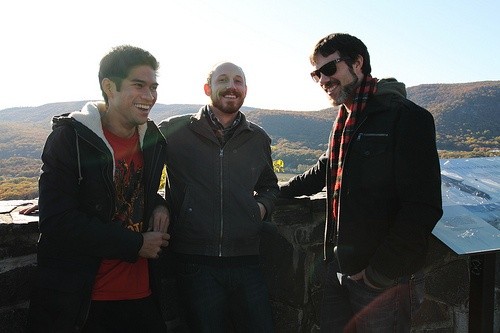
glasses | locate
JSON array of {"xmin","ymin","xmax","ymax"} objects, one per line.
[{"xmin": 310, "ymin": 56, "xmax": 344, "ymax": 82}]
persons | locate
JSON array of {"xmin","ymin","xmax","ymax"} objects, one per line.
[
  {"xmin": 20, "ymin": 62, "xmax": 280, "ymax": 333},
  {"xmin": 37, "ymin": 53, "xmax": 168, "ymax": 333},
  {"xmin": 280, "ymin": 33, "xmax": 444, "ymax": 333}
]
